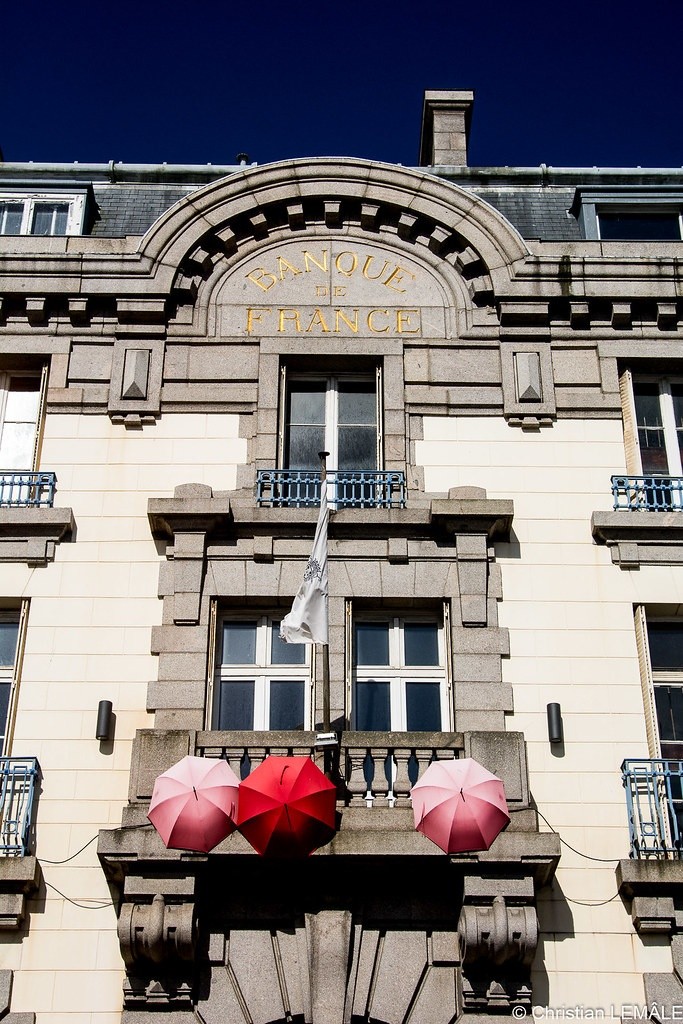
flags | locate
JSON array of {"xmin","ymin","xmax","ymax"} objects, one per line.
[{"xmin": 279, "ymin": 481, "xmax": 329, "ymax": 645}]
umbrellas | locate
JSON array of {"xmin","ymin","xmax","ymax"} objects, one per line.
[
  {"xmin": 237, "ymin": 755, "xmax": 342, "ymax": 859},
  {"xmin": 145, "ymin": 755, "xmax": 240, "ymax": 852},
  {"xmin": 410, "ymin": 757, "xmax": 510, "ymax": 853}
]
